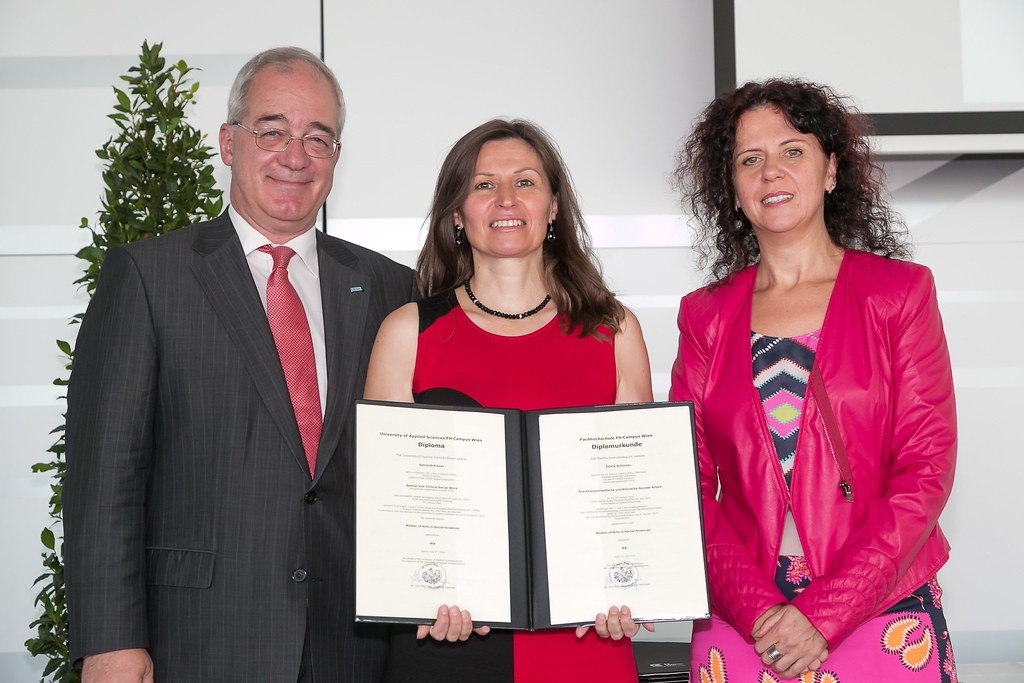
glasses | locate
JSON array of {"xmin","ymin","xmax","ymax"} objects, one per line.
[{"xmin": 233, "ymin": 121, "xmax": 342, "ymax": 159}]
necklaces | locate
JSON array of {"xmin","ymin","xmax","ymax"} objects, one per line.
[{"xmin": 462, "ymin": 273, "xmax": 551, "ymax": 319}]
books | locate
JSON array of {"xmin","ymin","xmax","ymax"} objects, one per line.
[{"xmin": 354, "ymin": 400, "xmax": 711, "ymax": 631}]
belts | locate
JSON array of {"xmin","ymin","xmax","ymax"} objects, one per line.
[{"xmin": 259, "ymin": 243, "xmax": 322, "ymax": 487}]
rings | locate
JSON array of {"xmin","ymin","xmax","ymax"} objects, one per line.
[{"xmin": 766, "ymin": 644, "xmax": 784, "ymax": 661}]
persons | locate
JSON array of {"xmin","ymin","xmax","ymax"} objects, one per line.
[
  {"xmin": 666, "ymin": 77, "xmax": 957, "ymax": 683},
  {"xmin": 61, "ymin": 47, "xmax": 655, "ymax": 683}
]
